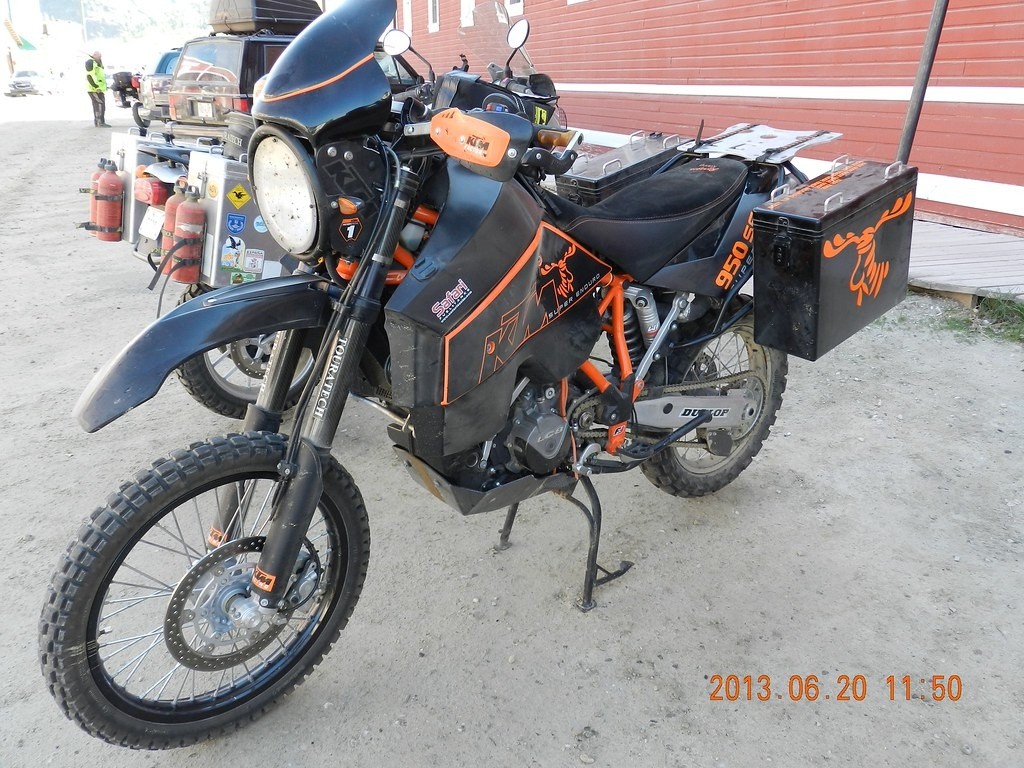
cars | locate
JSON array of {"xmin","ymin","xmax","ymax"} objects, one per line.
[{"xmin": 9, "ymin": 69, "xmax": 41, "ymax": 96}]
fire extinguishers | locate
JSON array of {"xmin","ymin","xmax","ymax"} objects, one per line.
[
  {"xmin": 171, "ymin": 185, "xmax": 206, "ymax": 284},
  {"xmin": 92, "ymin": 157, "xmax": 107, "ymax": 237},
  {"xmin": 161, "ymin": 179, "xmax": 189, "ymax": 275},
  {"xmin": 97, "ymin": 159, "xmax": 121, "ymax": 242}
]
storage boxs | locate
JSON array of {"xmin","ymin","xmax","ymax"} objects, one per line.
[
  {"xmin": 109, "ymin": 126, "xmax": 167, "ymax": 244},
  {"xmin": 553, "ymin": 129, "xmax": 695, "ymax": 207},
  {"xmin": 186, "ymin": 145, "xmax": 269, "ymax": 289},
  {"xmin": 208, "ymin": 0, "xmax": 323, "ymax": 35},
  {"xmin": 753, "ymin": 154, "xmax": 918, "ymax": 362}
]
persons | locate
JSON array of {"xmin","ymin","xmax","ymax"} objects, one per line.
[{"xmin": 85, "ymin": 51, "xmax": 112, "ymax": 129}]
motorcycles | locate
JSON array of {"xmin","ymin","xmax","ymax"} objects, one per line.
[{"xmin": 31, "ymin": 2, "xmax": 959, "ymax": 755}]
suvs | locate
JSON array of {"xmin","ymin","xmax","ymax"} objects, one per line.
[
  {"xmin": 134, "ymin": 47, "xmax": 182, "ymax": 136},
  {"xmin": 170, "ymin": 0, "xmax": 422, "ymax": 146}
]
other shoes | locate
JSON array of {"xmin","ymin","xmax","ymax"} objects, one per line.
[
  {"xmin": 99, "ymin": 123, "xmax": 111, "ymax": 127},
  {"xmin": 94, "ymin": 119, "xmax": 98, "ymax": 127}
]
